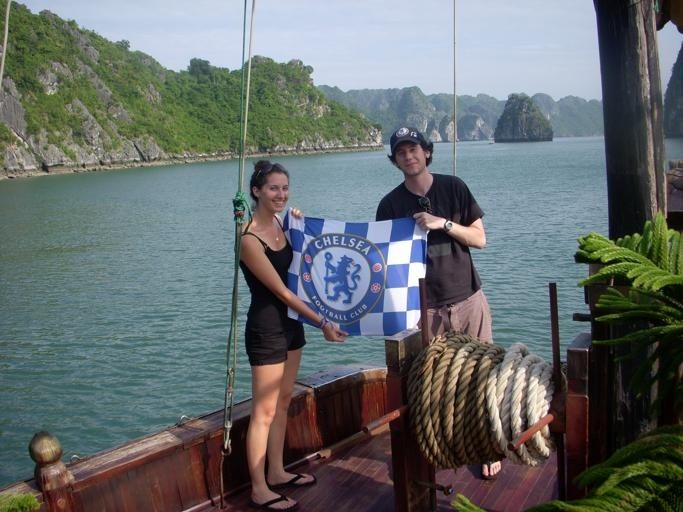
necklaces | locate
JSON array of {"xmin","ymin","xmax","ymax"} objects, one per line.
[{"xmin": 258, "ymin": 217, "xmax": 279, "ymax": 242}]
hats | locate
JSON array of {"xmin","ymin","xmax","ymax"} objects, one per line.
[{"xmin": 390, "ymin": 126, "xmax": 422, "ymax": 153}]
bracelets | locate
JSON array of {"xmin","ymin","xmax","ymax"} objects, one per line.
[{"xmin": 317, "ymin": 316, "xmax": 328, "ymax": 329}]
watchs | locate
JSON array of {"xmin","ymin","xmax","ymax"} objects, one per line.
[{"xmin": 443, "ymin": 218, "xmax": 452, "ymax": 232}]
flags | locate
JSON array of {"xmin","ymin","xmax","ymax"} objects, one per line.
[{"xmin": 278, "ymin": 205, "xmax": 428, "ymax": 342}]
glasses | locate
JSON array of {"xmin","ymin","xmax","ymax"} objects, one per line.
[
  {"xmin": 255, "ymin": 163, "xmax": 287, "ymax": 177},
  {"xmin": 418, "ymin": 196, "xmax": 432, "ymax": 214}
]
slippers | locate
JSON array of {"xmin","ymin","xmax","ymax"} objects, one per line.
[
  {"xmin": 267, "ymin": 472, "xmax": 316, "ymax": 490},
  {"xmin": 249, "ymin": 494, "xmax": 299, "ymax": 512},
  {"xmin": 480, "ymin": 460, "xmax": 500, "ymax": 480}
]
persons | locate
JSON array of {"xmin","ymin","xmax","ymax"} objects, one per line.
[
  {"xmin": 370, "ymin": 124, "xmax": 502, "ymax": 481},
  {"xmin": 228, "ymin": 159, "xmax": 318, "ymax": 512}
]
